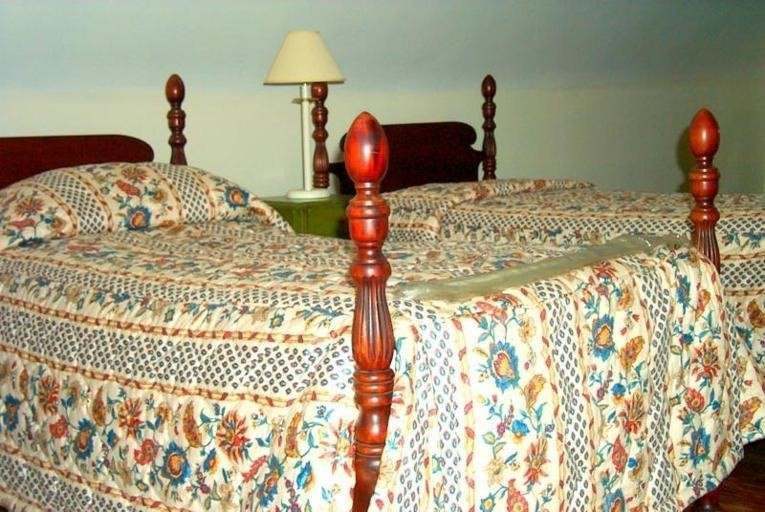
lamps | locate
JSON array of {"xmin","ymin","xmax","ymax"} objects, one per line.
[{"xmin": 266, "ymin": 26, "xmax": 345, "ymax": 201}]
